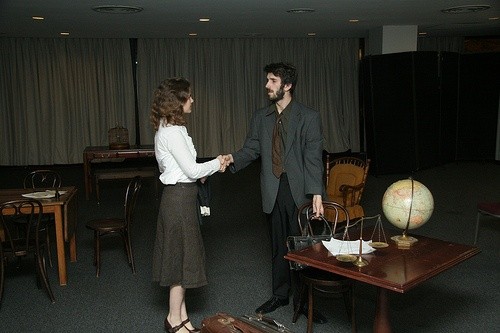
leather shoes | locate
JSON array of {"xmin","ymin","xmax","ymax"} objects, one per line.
[
  {"xmin": 255, "ymin": 296, "xmax": 289, "ymax": 315},
  {"xmin": 294, "ymin": 303, "xmax": 327, "ymax": 324}
]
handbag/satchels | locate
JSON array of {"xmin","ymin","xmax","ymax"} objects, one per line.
[{"xmin": 287, "ymin": 213, "xmax": 344, "ymax": 270}]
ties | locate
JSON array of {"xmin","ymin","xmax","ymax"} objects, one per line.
[{"xmin": 272, "ymin": 116, "xmax": 283, "ymax": 179}]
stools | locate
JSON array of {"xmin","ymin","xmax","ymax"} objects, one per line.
[{"xmin": 474, "ymin": 201, "xmax": 500, "ymax": 246}]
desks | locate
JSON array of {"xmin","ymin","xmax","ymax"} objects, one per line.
[
  {"xmin": 284, "ymin": 228, "xmax": 481, "ymax": 333},
  {"xmin": 0, "ymin": 186, "xmax": 78, "ymax": 285},
  {"xmin": 84, "ymin": 145, "xmax": 165, "ymax": 201}
]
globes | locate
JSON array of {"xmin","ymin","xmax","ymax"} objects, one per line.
[{"xmin": 382, "ymin": 176, "xmax": 434, "ymax": 244}]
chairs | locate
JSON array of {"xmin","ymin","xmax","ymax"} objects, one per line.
[
  {"xmin": 292, "ymin": 200, "xmax": 360, "ymax": 333},
  {"xmin": 10, "ymin": 169, "xmax": 62, "ymax": 270},
  {"xmin": 323, "ymin": 153, "xmax": 370, "ymax": 235},
  {"xmin": 0, "ymin": 198, "xmax": 56, "ymax": 307},
  {"xmin": 86, "ymin": 175, "xmax": 143, "ymax": 278}
]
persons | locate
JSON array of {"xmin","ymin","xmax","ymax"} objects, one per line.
[
  {"xmin": 151, "ymin": 78, "xmax": 227, "ymax": 333},
  {"xmin": 220, "ymin": 63, "xmax": 328, "ymax": 324}
]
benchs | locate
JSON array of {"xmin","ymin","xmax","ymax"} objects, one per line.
[{"xmin": 94, "ymin": 166, "xmax": 160, "ymax": 204}]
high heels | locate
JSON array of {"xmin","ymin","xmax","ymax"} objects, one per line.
[{"xmin": 164, "ymin": 314, "xmax": 201, "ymax": 333}]
